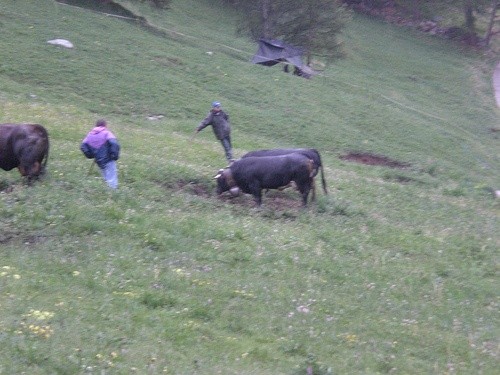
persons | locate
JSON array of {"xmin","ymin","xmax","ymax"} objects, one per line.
[
  {"xmin": 195, "ymin": 101, "xmax": 236, "ymax": 162},
  {"xmin": 79, "ymin": 119, "xmax": 120, "ymax": 189}
]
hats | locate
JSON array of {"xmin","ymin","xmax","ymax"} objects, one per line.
[{"xmin": 213, "ymin": 102, "xmax": 222, "ymax": 107}]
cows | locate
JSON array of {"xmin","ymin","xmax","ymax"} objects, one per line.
[
  {"xmin": 226, "ymin": 149, "xmax": 327, "ymax": 202},
  {"xmin": 0, "ymin": 124, "xmax": 48, "ymax": 187},
  {"xmin": 212, "ymin": 154, "xmax": 319, "ymax": 209}
]
